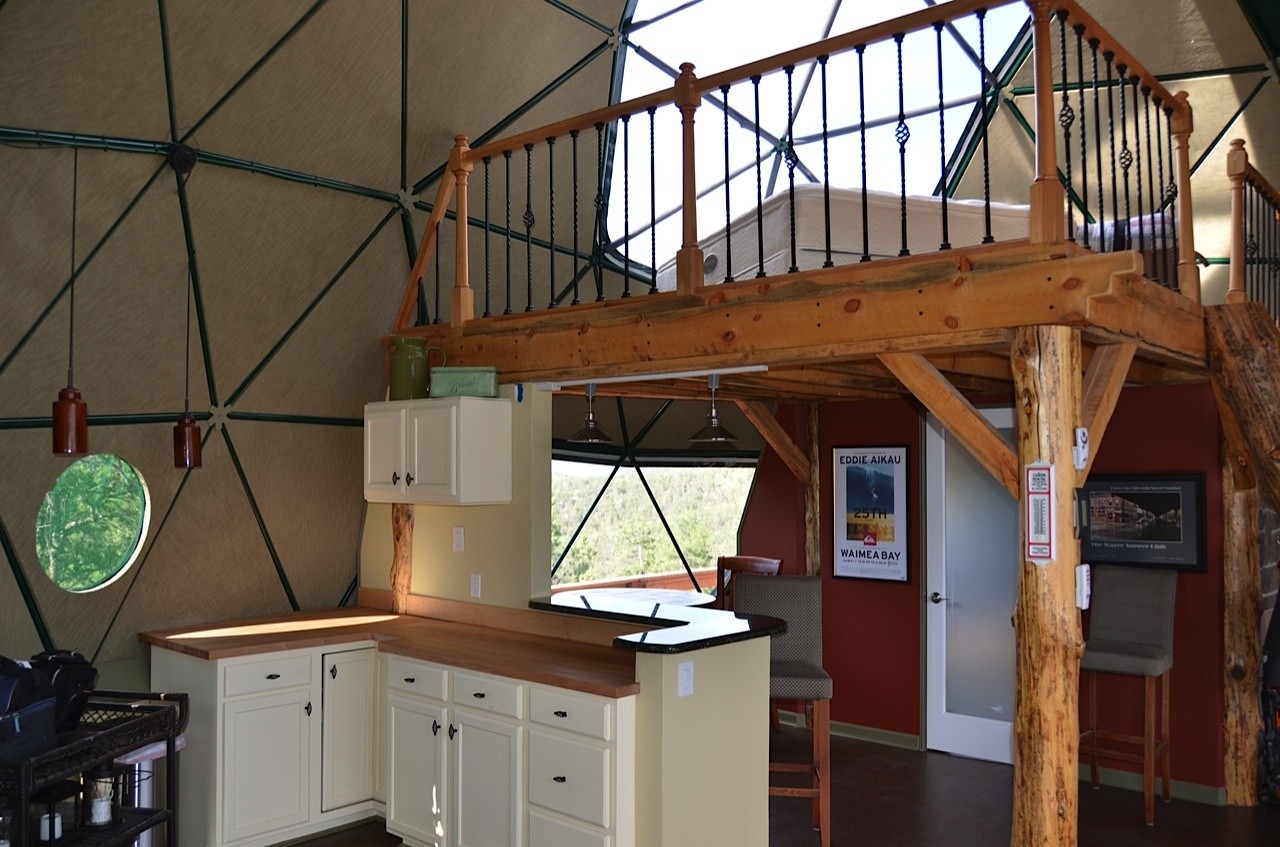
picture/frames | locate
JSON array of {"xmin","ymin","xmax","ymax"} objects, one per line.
[
  {"xmin": 830, "ymin": 442, "xmax": 911, "ymax": 584},
  {"xmin": 1078, "ymin": 470, "xmax": 1208, "ymax": 574}
]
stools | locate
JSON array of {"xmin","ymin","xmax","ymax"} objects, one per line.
[
  {"xmin": 1079, "ymin": 564, "xmax": 1179, "ymax": 825},
  {"xmin": 717, "ymin": 556, "xmax": 832, "ymax": 847}
]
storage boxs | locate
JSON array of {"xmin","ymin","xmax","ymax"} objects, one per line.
[{"xmin": 431, "ymin": 366, "xmax": 499, "ymax": 398}]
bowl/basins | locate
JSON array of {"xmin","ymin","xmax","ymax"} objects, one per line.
[{"xmin": 429, "ymin": 365, "xmax": 500, "ymax": 397}]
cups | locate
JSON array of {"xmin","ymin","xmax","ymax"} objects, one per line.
[
  {"xmin": 40, "ymin": 813, "xmax": 62, "ymax": 841},
  {"xmin": 92, "ymin": 798, "xmax": 111, "ymax": 823}
]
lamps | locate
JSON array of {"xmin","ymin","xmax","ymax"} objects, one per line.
[
  {"xmin": 691, "ymin": 375, "xmax": 740, "ymax": 442},
  {"xmin": 569, "ymin": 383, "xmax": 613, "ymax": 443},
  {"xmin": 52, "ymin": 147, "xmax": 88, "ymax": 458},
  {"xmin": 172, "ymin": 148, "xmax": 201, "ymax": 469}
]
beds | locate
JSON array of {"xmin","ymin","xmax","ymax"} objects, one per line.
[{"xmin": 656, "ymin": 182, "xmax": 1077, "ymax": 293}]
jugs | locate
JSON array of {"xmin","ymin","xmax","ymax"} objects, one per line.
[{"xmin": 388, "ymin": 337, "xmax": 446, "ymax": 401}]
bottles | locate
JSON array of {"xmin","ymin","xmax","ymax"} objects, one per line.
[
  {"xmin": 52, "ymin": 391, "xmax": 88, "ymax": 455},
  {"xmin": 173, "ymin": 416, "xmax": 202, "ymax": 468}
]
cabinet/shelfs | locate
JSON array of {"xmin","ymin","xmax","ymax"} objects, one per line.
[{"xmin": 0, "ymin": 381, "xmax": 786, "ymax": 847}]
